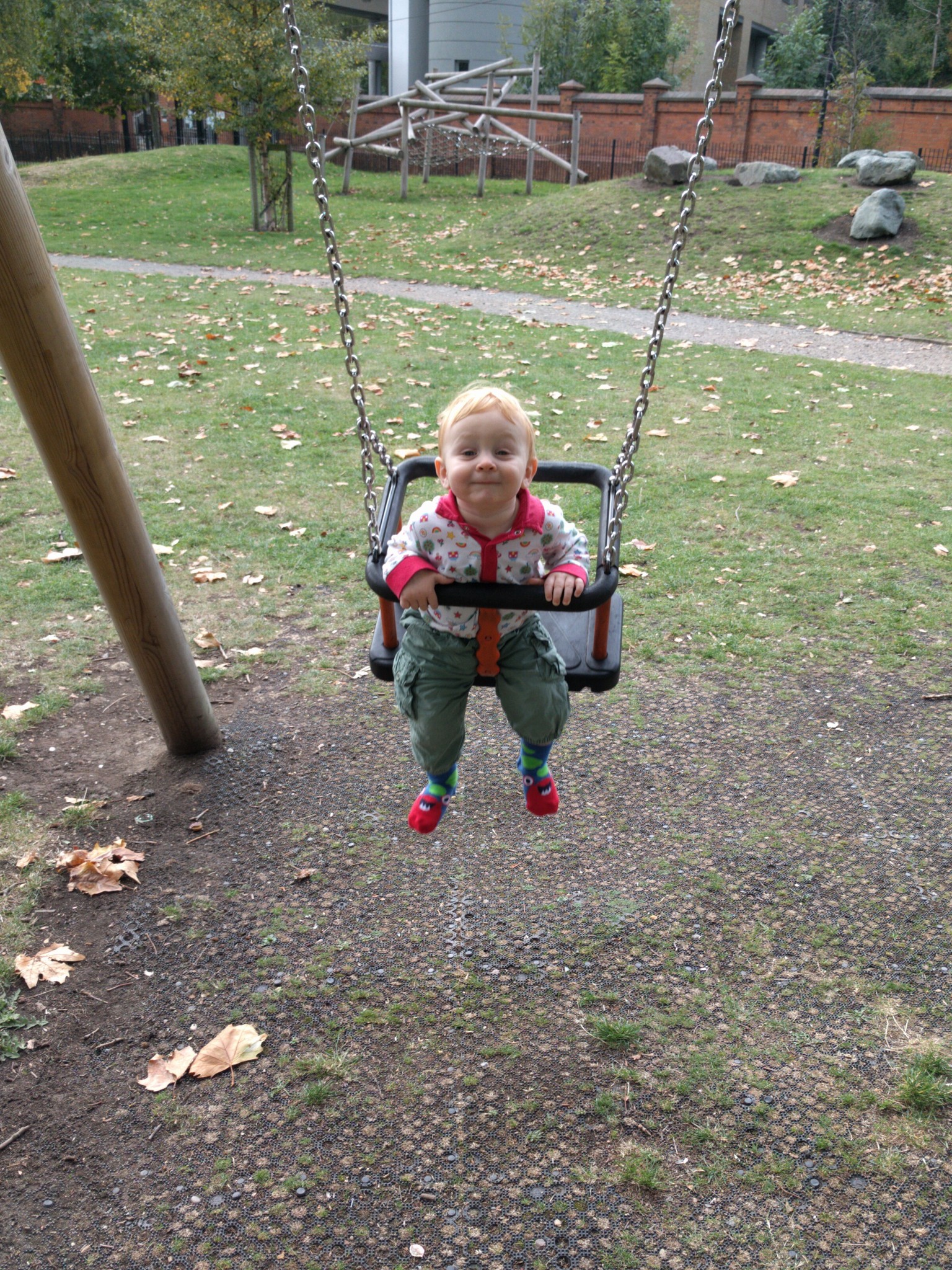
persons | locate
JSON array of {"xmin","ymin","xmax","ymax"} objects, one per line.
[{"xmin": 380, "ymin": 381, "xmax": 588, "ymax": 833}]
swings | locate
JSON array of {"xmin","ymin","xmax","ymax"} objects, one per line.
[{"xmin": 279, "ymin": 0, "xmax": 742, "ymax": 694}]
